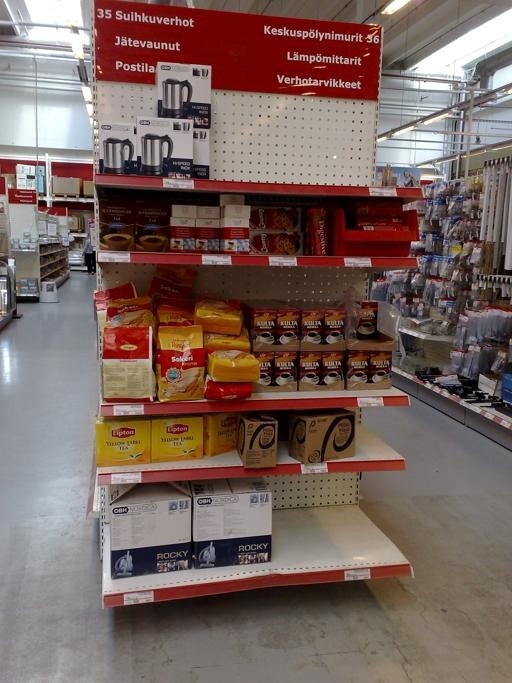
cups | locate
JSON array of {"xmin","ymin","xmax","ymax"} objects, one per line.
[
  {"xmin": 139, "ymin": 235, "xmax": 168, "ymax": 252},
  {"xmin": 103, "ymin": 233, "xmax": 132, "ymax": 250},
  {"xmin": 361, "ymin": 321, "xmax": 375, "ymax": 330},
  {"xmin": 259, "ymin": 330, "xmax": 343, "ymax": 342},
  {"xmin": 258, "ymin": 372, "xmax": 339, "ymax": 383},
  {"xmin": 352, "ymin": 371, "xmax": 388, "ymax": 381}
]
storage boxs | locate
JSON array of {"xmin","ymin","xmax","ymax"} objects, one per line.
[
  {"xmin": 94, "ymin": 414, "xmax": 240, "ymax": 467},
  {"xmin": 287, "ymin": 409, "xmax": 356, "ymax": 463},
  {"xmin": 99, "ymin": 61, "xmax": 211, "ymax": 179},
  {"xmin": 190, "ymin": 482, "xmax": 271, "ymax": 568},
  {"xmin": 238, "ymin": 413, "xmax": 279, "ymax": 469},
  {"xmin": 53, "ymin": 176, "xmax": 82, "ymax": 198},
  {"xmin": 107, "ymin": 482, "xmax": 192, "ymax": 580}
]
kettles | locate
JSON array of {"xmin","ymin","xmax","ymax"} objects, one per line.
[
  {"xmin": 161, "ymin": 79, "xmax": 192, "ymax": 118},
  {"xmin": 102, "ymin": 137, "xmax": 134, "ymax": 174},
  {"xmin": 140, "ymin": 133, "xmax": 174, "ymax": 176}
]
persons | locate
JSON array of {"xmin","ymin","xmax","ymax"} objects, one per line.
[
  {"xmin": 404, "ymin": 170, "xmax": 418, "ymax": 186},
  {"xmin": 82, "ymin": 233, "xmax": 95, "ymax": 273}
]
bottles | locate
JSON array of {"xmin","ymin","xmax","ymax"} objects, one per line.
[{"xmin": 306, "ymin": 207, "xmax": 330, "ymax": 256}]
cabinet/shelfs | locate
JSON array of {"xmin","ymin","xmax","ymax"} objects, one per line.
[
  {"xmin": 98, "ymin": 175, "xmax": 413, "ymax": 608},
  {"xmin": 14, "ymin": 241, "xmax": 71, "ymax": 300}
]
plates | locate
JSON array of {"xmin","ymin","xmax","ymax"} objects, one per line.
[
  {"xmin": 350, "ymin": 376, "xmax": 376, "ymax": 383},
  {"xmin": 265, "ymin": 376, "xmax": 335, "ymax": 386},
  {"xmin": 358, "ymin": 328, "xmax": 375, "ymax": 335},
  {"xmin": 256, "ymin": 334, "xmax": 334, "ymax": 344}
]
